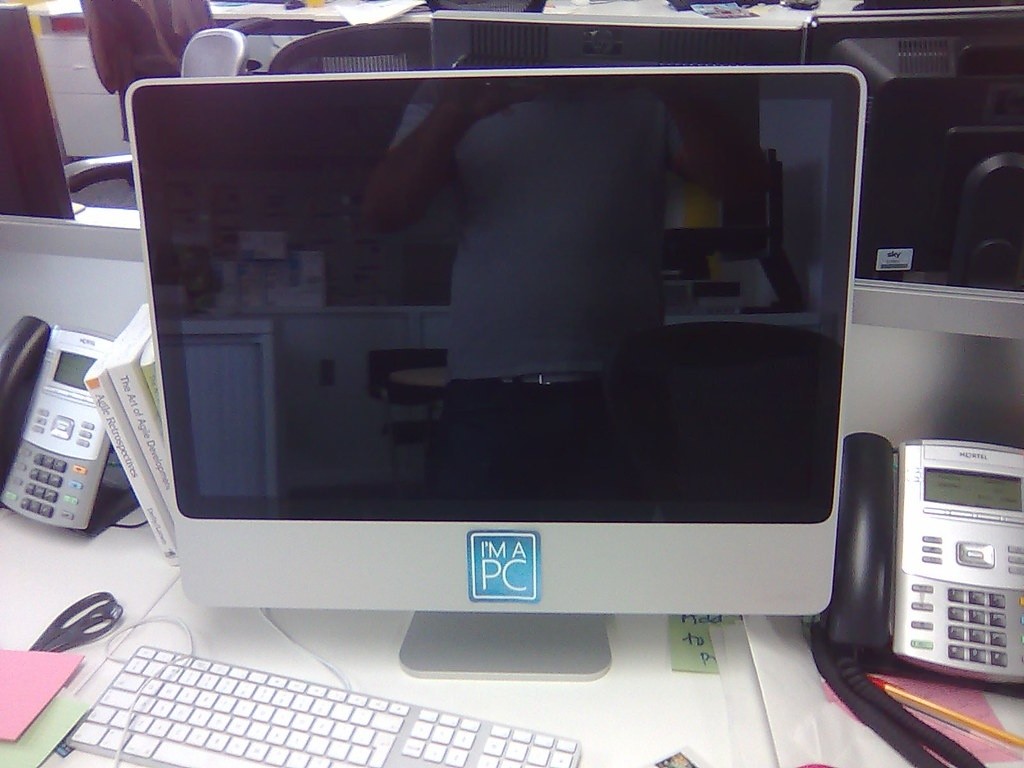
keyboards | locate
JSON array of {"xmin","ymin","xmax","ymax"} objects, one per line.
[{"xmin": 66, "ymin": 646, "xmax": 581, "ymax": 767}]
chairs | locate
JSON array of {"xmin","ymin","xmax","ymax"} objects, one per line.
[
  {"xmin": 80, "ymin": 0, "xmax": 267, "ymax": 142},
  {"xmin": 267, "ymin": 22, "xmax": 434, "ymax": 78},
  {"xmin": 63, "ymin": 29, "xmax": 248, "ymax": 194},
  {"xmin": 426, "ymin": 0, "xmax": 546, "ymax": 13}
]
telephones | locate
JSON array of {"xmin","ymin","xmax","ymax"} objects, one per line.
[
  {"xmin": 820, "ymin": 432, "xmax": 1024, "ymax": 687},
  {"xmin": 1, "ymin": 315, "xmax": 118, "ymax": 533}
]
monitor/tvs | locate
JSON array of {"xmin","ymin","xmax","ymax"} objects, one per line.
[
  {"xmin": 0, "ymin": 6, "xmax": 75, "ymax": 221},
  {"xmin": 125, "ymin": 5, "xmax": 1024, "ymax": 684}
]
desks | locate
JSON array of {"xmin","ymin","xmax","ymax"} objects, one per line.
[
  {"xmin": 0, "ymin": 451, "xmax": 182, "ymax": 768},
  {"xmin": 34, "ymin": 567, "xmax": 1024, "ymax": 768}
]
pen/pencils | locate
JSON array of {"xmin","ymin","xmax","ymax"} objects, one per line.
[{"xmin": 866, "ymin": 672, "xmax": 1024, "ymax": 757}]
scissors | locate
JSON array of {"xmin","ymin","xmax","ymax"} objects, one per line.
[{"xmin": 30, "ymin": 591, "xmax": 123, "ymax": 654}]
